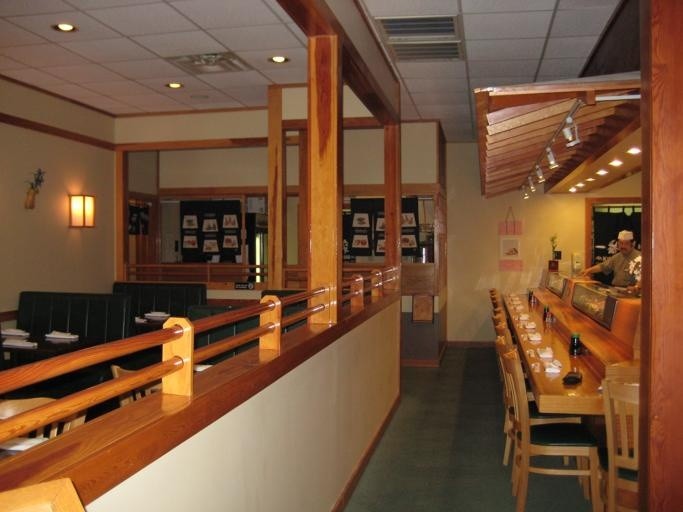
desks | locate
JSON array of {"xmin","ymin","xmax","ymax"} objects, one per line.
[{"xmin": 0, "ymin": 438, "xmax": 49, "ymax": 462}]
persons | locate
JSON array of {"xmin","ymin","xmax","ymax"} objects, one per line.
[{"xmin": 577, "ymin": 229, "xmax": 642, "ymax": 297}]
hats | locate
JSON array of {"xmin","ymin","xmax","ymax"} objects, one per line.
[{"xmin": 618, "ymin": 230, "xmax": 633, "ymax": 241}]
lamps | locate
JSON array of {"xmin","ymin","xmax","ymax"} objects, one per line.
[
  {"xmin": 519, "ymin": 100, "xmax": 585, "ymax": 199},
  {"xmin": 69, "ymin": 194, "xmax": 96, "ymax": 227}
]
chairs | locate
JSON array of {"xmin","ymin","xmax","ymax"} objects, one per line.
[
  {"xmin": 490, "ymin": 288, "xmax": 640, "ymax": 512},
  {"xmin": 0, "ymin": 365, "xmax": 163, "ymax": 438}
]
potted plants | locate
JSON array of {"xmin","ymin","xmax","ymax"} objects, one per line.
[
  {"xmin": 25, "ymin": 169, "xmax": 46, "ymax": 208},
  {"xmin": 548, "ymin": 235, "xmax": 559, "ymax": 272}
]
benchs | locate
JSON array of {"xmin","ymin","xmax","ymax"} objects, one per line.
[{"xmin": 16, "ymin": 280, "xmax": 308, "ymax": 364}]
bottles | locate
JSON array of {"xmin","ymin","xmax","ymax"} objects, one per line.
[
  {"xmin": 529, "ymin": 287, "xmax": 533, "ymax": 302},
  {"xmin": 569, "ymin": 334, "xmax": 580, "ymax": 355},
  {"xmin": 543, "ymin": 304, "xmax": 551, "ymax": 321}
]
